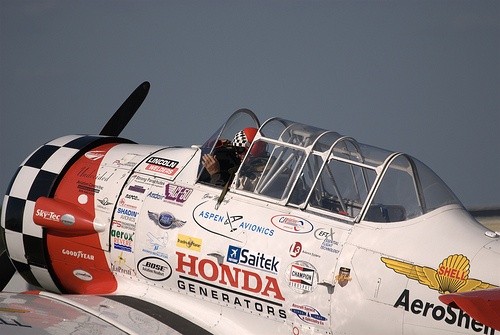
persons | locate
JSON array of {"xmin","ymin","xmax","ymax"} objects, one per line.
[{"xmin": 201, "ymin": 127, "xmax": 293, "ymax": 194}]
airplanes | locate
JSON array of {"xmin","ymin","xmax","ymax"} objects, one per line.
[{"xmin": 1, "ymin": 80, "xmax": 499, "ymax": 334}]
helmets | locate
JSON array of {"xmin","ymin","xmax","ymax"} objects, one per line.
[{"xmin": 231, "ymin": 127, "xmax": 267, "ymax": 161}]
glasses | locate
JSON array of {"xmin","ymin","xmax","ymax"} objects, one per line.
[{"xmin": 232, "ymin": 146, "xmax": 246, "ymax": 154}]
